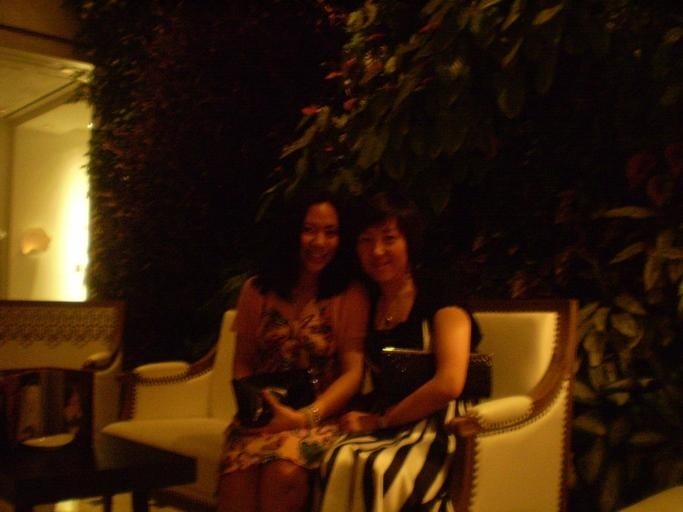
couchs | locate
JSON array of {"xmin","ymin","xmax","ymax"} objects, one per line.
[{"xmin": 103, "ymin": 292, "xmax": 580, "ymax": 512}]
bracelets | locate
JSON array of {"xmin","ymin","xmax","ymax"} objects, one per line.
[
  {"xmin": 378, "ymin": 409, "xmax": 386, "ymax": 430},
  {"xmin": 308, "ymin": 402, "xmax": 320, "ymax": 426}
]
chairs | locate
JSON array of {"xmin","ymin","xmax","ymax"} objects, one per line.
[{"xmin": 0, "ymin": 299, "xmax": 128, "ymax": 512}]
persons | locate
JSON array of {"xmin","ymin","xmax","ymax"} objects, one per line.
[
  {"xmin": 310, "ymin": 196, "xmax": 490, "ymax": 511},
  {"xmin": 212, "ymin": 188, "xmax": 373, "ymax": 511}
]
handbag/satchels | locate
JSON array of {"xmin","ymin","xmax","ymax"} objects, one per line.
[
  {"xmin": 230, "ymin": 363, "xmax": 315, "ymax": 428},
  {"xmin": 370, "ymin": 324, "xmax": 490, "ymax": 408}
]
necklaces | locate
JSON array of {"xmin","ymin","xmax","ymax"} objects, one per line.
[{"xmin": 379, "ymin": 297, "xmax": 400, "ymax": 325}]
